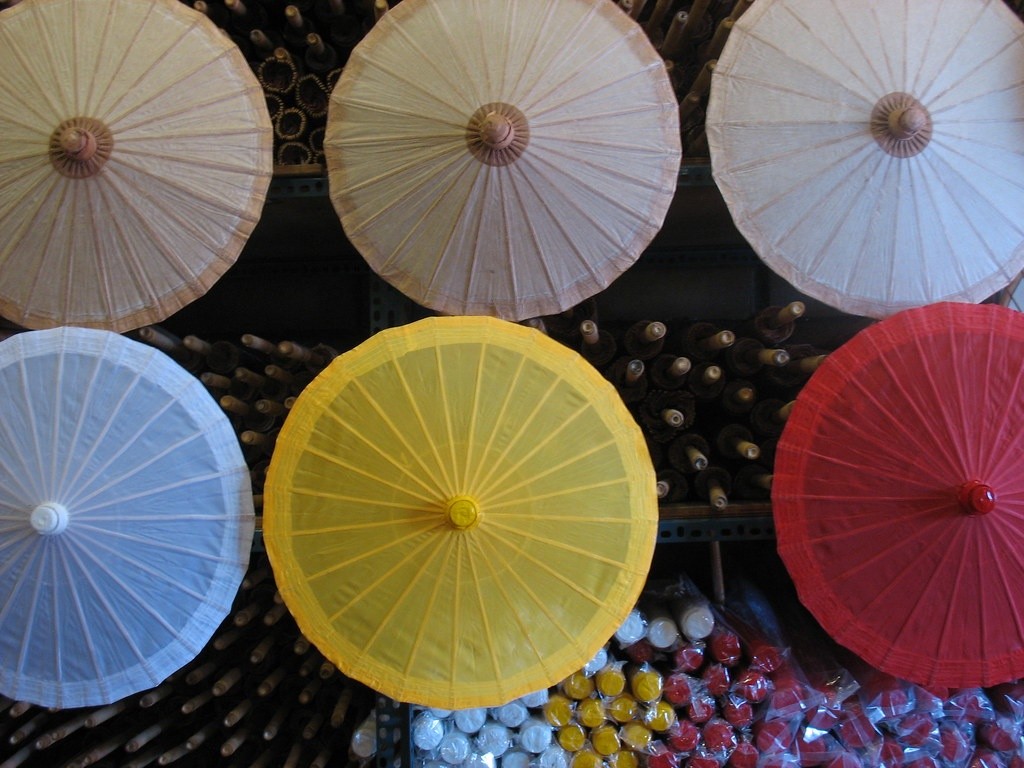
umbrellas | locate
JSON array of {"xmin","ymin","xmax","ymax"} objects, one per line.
[
  {"xmin": 772, "ymin": 303, "xmax": 1023, "ymax": 687},
  {"xmin": 0, "ymin": 0, "xmax": 274, "ymax": 335},
  {"xmin": 263, "ymin": 316, "xmax": 660, "ymax": 711},
  {"xmin": 0, "ymin": 0, "xmax": 1024, "ymax": 767},
  {"xmin": 325, "ymin": 0, "xmax": 683, "ymax": 322},
  {"xmin": 705, "ymin": 0, "xmax": 1024, "ymax": 321},
  {"xmin": 0, "ymin": 327, "xmax": 255, "ymax": 711}
]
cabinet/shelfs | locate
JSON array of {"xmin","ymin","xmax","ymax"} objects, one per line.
[{"xmin": 0, "ymin": 0, "xmax": 1023, "ymax": 768}]
